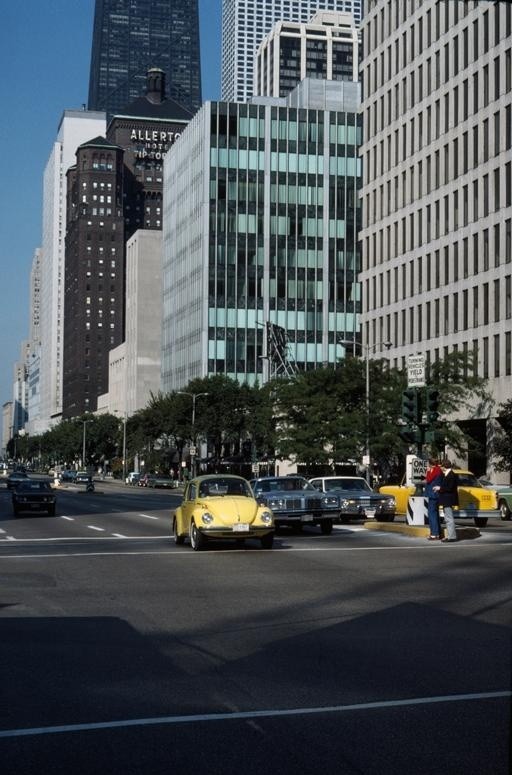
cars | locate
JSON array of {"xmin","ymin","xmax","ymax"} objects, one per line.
[
  {"xmin": 303, "ymin": 475, "xmax": 397, "ymax": 524},
  {"xmin": 172, "ymin": 474, "xmax": 275, "ymax": 550},
  {"xmin": 245, "ymin": 475, "xmax": 342, "ymax": 535},
  {"xmin": 8, "ymin": 469, "xmax": 172, "ymax": 516},
  {"xmin": 495, "ymin": 484, "xmax": 512, "ymax": 522},
  {"xmin": 379, "ymin": 469, "xmax": 502, "ymax": 527}
]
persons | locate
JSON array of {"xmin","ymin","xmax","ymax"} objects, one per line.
[
  {"xmin": 432, "ymin": 459, "xmax": 460, "ymax": 543},
  {"xmin": 424, "ymin": 457, "xmax": 443, "ymax": 541}
]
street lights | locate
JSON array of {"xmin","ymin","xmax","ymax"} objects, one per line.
[
  {"xmin": 338, "ymin": 340, "xmax": 392, "ymax": 483},
  {"xmin": 175, "ymin": 391, "xmax": 211, "ymax": 477},
  {"xmin": 114, "ymin": 409, "xmax": 138, "ymax": 478},
  {"xmin": 77, "ymin": 419, "xmax": 94, "ymax": 465}
]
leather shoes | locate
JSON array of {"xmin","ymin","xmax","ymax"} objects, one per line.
[{"xmin": 427, "ymin": 535, "xmax": 456, "ymax": 542}]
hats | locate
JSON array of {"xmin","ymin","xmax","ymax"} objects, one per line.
[{"xmin": 438, "ymin": 460, "xmax": 451, "ymax": 467}]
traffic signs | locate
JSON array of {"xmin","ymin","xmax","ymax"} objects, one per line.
[{"xmin": 412, "ymin": 458, "xmax": 430, "ymax": 484}]
